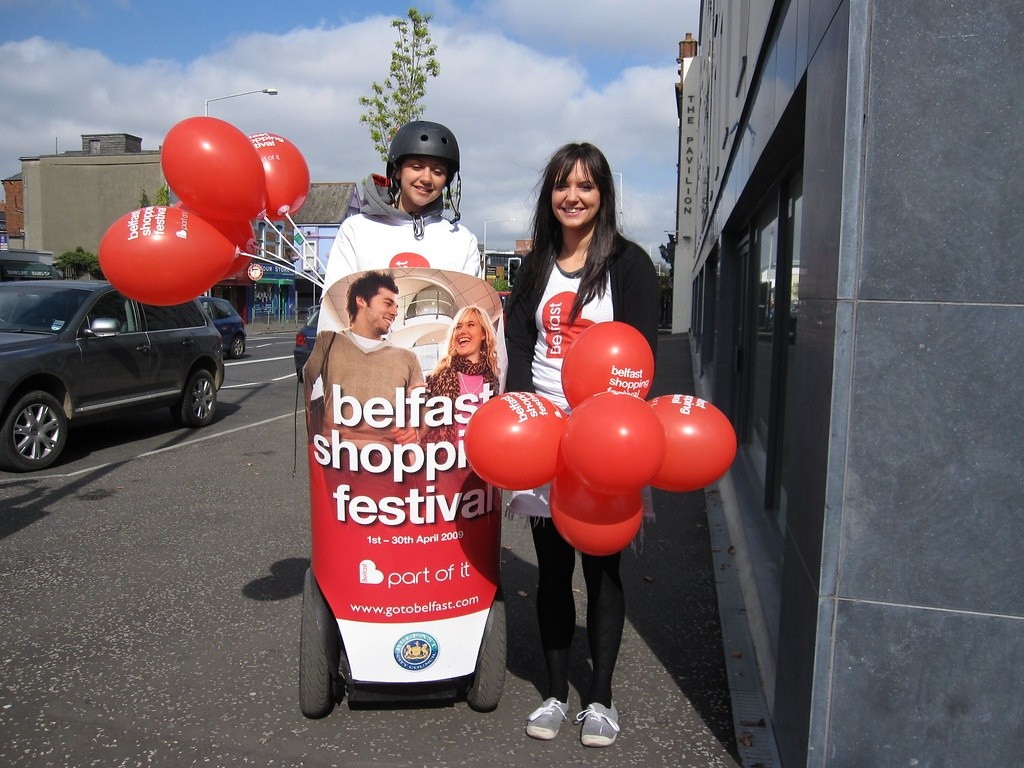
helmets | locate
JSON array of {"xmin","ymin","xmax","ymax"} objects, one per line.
[{"xmin": 386, "ymin": 121, "xmax": 461, "ymax": 175}]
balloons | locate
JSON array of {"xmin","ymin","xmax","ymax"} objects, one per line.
[
  {"xmin": 99, "ymin": 116, "xmax": 310, "ymax": 307},
  {"xmin": 463, "ymin": 321, "xmax": 737, "ymax": 557}
]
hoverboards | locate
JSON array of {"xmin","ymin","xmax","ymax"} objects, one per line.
[{"xmin": 298, "ymin": 267, "xmax": 510, "ymax": 719}]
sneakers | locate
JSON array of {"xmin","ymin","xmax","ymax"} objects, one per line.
[
  {"xmin": 527, "ymin": 693, "xmax": 570, "ymax": 739},
  {"xmin": 576, "ymin": 699, "xmax": 620, "ymax": 748}
]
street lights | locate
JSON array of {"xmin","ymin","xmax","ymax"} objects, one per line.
[{"xmin": 204, "ymin": 88, "xmax": 278, "ymax": 117}]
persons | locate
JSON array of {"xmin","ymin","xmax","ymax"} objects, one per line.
[
  {"xmin": 301, "ymin": 271, "xmax": 432, "ymax": 448},
  {"xmin": 305, "ymin": 121, "xmax": 501, "ymax": 450},
  {"xmin": 504, "ymin": 142, "xmax": 660, "ymax": 747},
  {"xmin": 406, "ymin": 304, "xmax": 501, "ymax": 462}
]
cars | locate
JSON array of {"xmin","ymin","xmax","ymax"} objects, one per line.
[
  {"xmin": 198, "ymin": 296, "xmax": 246, "ymax": 359},
  {"xmin": 0, "ymin": 279, "xmax": 225, "ymax": 473},
  {"xmin": 293, "ymin": 311, "xmax": 320, "ymax": 383}
]
